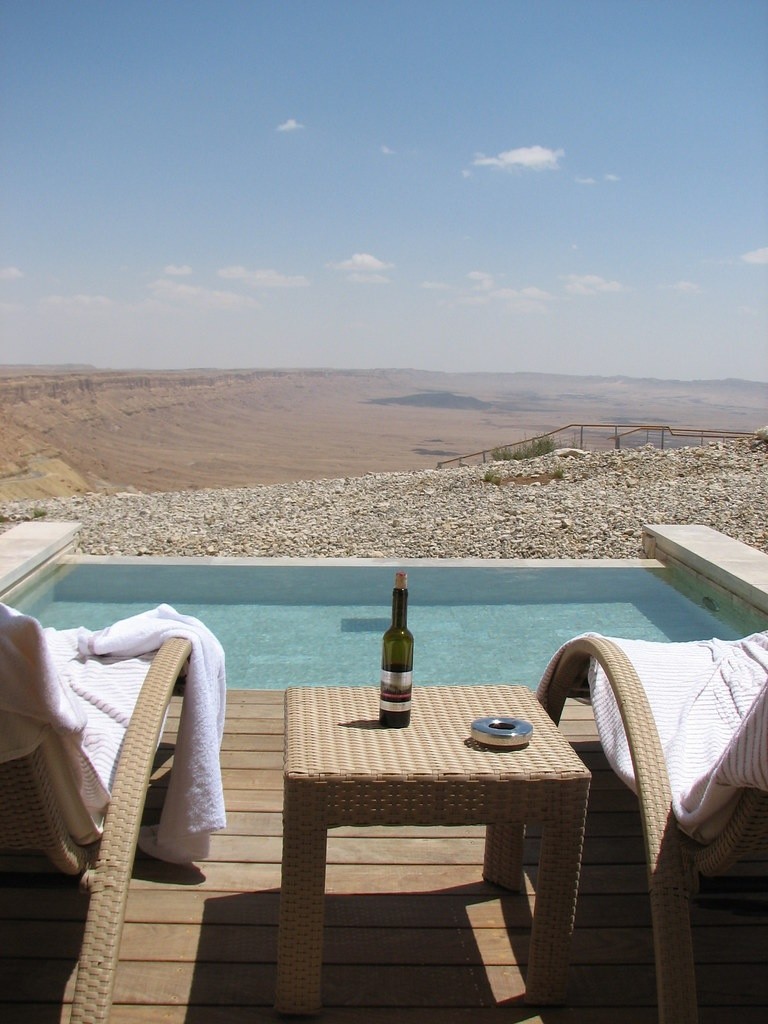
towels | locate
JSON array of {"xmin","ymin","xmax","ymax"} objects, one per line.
[
  {"xmin": 0, "ymin": 592, "xmax": 235, "ymax": 863},
  {"xmin": 533, "ymin": 622, "xmax": 768, "ymax": 844}
]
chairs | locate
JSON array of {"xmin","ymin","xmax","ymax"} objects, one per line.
[
  {"xmin": 0, "ymin": 600, "xmax": 229, "ymax": 1024},
  {"xmin": 537, "ymin": 629, "xmax": 768, "ymax": 1024}
]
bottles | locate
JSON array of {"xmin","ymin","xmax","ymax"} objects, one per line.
[{"xmin": 379, "ymin": 572, "xmax": 414, "ymax": 729}]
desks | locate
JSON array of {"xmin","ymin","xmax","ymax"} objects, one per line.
[{"xmin": 273, "ymin": 684, "xmax": 592, "ymax": 1015}]
